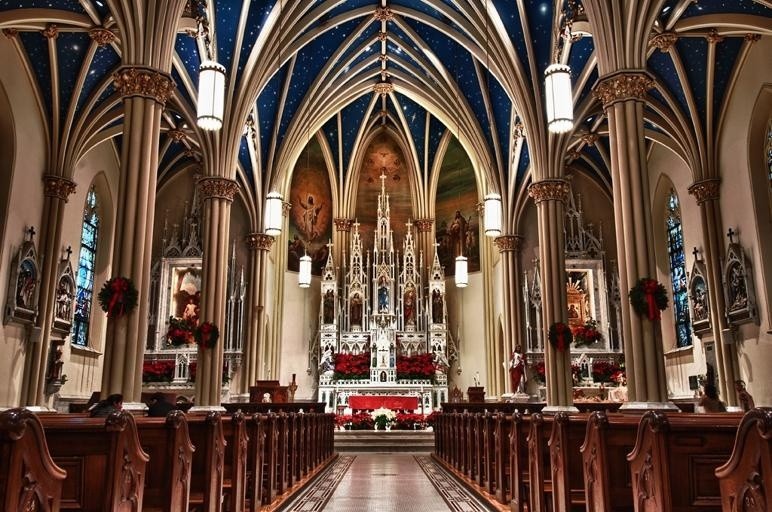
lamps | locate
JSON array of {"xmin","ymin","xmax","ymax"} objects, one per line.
[
  {"xmin": 196, "ymin": 0, "xmax": 227, "ymax": 132},
  {"xmin": 263, "ymin": 0, "xmax": 313, "ymax": 289},
  {"xmin": 454, "ymin": 1, "xmax": 502, "ymax": 288},
  {"xmin": 543, "ymin": 1, "xmax": 575, "ymax": 134}
]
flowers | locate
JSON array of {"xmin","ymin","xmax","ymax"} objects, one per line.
[
  {"xmin": 335, "ymin": 411, "xmax": 440, "ymax": 427},
  {"xmin": 333, "ymin": 352, "xmax": 440, "ymax": 386}
]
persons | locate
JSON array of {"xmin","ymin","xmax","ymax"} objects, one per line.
[
  {"xmin": 432, "ymin": 288, "xmax": 442, "ymax": 323},
  {"xmin": 89, "ymin": 393, "xmax": 125, "ymax": 417},
  {"xmin": 697, "ymin": 384, "xmax": 724, "ymax": 414},
  {"xmin": 507, "ymin": 344, "xmax": 526, "ymax": 393},
  {"xmin": 324, "ymin": 289, "xmax": 334, "ymax": 324},
  {"xmin": 349, "ymin": 293, "xmax": 363, "ymax": 326},
  {"xmin": 184, "ymin": 298, "xmax": 199, "ymax": 323},
  {"xmin": 290, "ymin": 193, "xmax": 329, "ymax": 271},
  {"xmin": 438, "ymin": 209, "xmax": 478, "ymax": 263},
  {"xmin": 377, "ymin": 277, "xmax": 389, "ymax": 311},
  {"xmin": 404, "ymin": 287, "xmax": 417, "ymax": 324},
  {"xmin": 733, "ymin": 379, "xmax": 755, "ymax": 411},
  {"xmin": 149, "ymin": 392, "xmax": 178, "ymax": 416}
]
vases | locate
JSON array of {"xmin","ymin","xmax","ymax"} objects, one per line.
[{"xmin": 345, "ymin": 424, "xmax": 427, "ymax": 430}]
